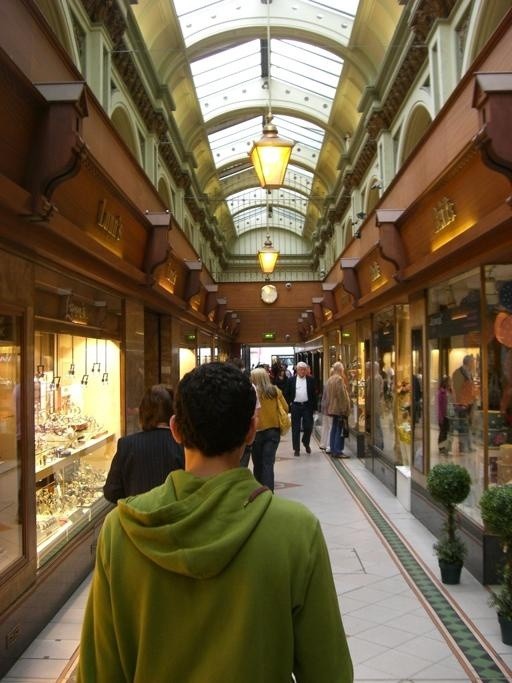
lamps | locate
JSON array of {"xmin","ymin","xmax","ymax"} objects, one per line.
[
  {"xmin": 247, "ymin": 0, "xmax": 299, "ymax": 191},
  {"xmin": 256, "ymin": 188, "xmax": 281, "ymax": 284}
]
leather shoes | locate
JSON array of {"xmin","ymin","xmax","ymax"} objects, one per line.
[
  {"xmin": 295, "ymin": 450, "xmax": 299, "ymax": 456},
  {"xmin": 303, "ymin": 442, "xmax": 310, "ymax": 452},
  {"xmin": 332, "ymin": 452, "xmax": 349, "ymax": 458}
]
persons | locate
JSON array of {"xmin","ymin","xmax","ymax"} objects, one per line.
[
  {"xmin": 78, "ymin": 361, "xmax": 355, "ymax": 683},
  {"xmin": 362, "ymin": 352, "xmax": 479, "ymax": 455},
  {"xmin": 225, "ymin": 356, "xmax": 354, "ymax": 497},
  {"xmin": 104, "ymin": 383, "xmax": 184, "ymax": 508}
]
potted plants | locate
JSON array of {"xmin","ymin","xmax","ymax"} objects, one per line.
[
  {"xmin": 425, "ymin": 461, "xmax": 475, "ymax": 587},
  {"xmin": 477, "ymin": 481, "xmax": 512, "ymax": 646}
]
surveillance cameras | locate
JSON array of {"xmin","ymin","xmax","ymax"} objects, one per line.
[{"xmin": 286, "ymin": 282, "xmax": 291, "ymax": 288}]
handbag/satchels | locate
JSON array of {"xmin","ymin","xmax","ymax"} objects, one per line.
[{"xmin": 278, "ymin": 401, "xmax": 291, "ymax": 436}]
